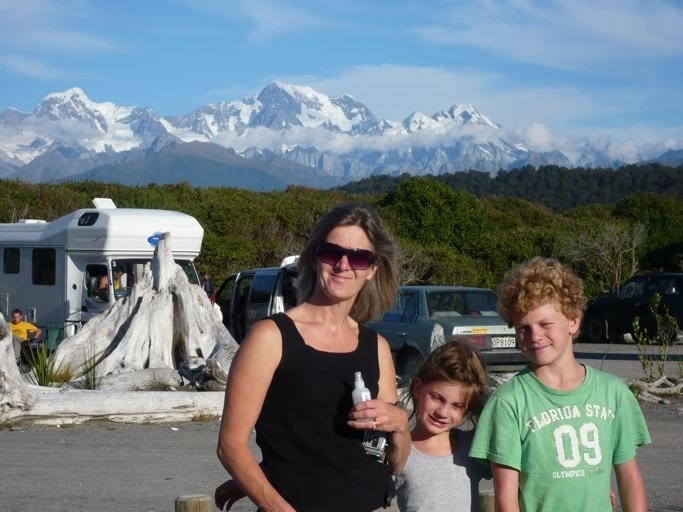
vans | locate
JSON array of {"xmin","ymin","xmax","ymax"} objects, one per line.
[
  {"xmin": 209, "ymin": 267, "xmax": 298, "ymax": 343},
  {"xmin": 583, "ymin": 274, "xmax": 682, "ymax": 344}
]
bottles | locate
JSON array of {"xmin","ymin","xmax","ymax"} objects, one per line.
[{"xmin": 352, "ymin": 371, "xmax": 374, "ymax": 424}]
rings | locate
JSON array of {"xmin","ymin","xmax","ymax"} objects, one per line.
[
  {"xmin": 373, "ymin": 422, "xmax": 382, "ymax": 430},
  {"xmin": 372, "ymin": 416, "xmax": 376, "ymax": 421}
]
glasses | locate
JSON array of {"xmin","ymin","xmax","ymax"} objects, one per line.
[{"xmin": 316, "ymin": 238, "xmax": 376, "ymax": 272}]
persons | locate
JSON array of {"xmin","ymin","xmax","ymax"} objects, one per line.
[
  {"xmin": 466, "ymin": 254, "xmax": 651, "ymax": 512},
  {"xmin": 97, "ymin": 265, "xmax": 124, "ymax": 290},
  {"xmin": 0, "ymin": 308, "xmax": 41, "ymax": 364},
  {"xmin": 216, "ymin": 205, "xmax": 411, "ymax": 512},
  {"xmin": 384, "ymin": 339, "xmax": 493, "ymax": 512}
]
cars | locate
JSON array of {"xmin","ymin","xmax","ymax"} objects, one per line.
[{"xmin": 364, "ymin": 285, "xmax": 530, "ymax": 375}]
